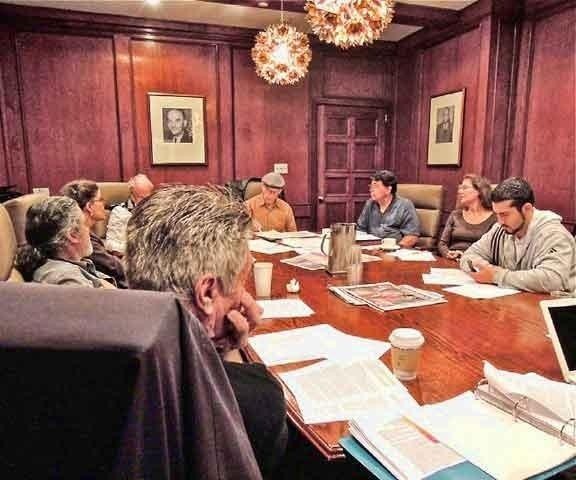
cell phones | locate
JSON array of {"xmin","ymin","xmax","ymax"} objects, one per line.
[{"xmin": 467, "ymin": 259, "xmax": 478, "ymax": 272}]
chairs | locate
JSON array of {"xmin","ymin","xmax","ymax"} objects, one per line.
[
  {"xmin": 0, "ymin": 194, "xmax": 49, "ymax": 283},
  {"xmin": 93, "ymin": 182, "xmax": 132, "ymax": 238},
  {"xmin": 398, "ymin": 184, "xmax": 442, "ymax": 250},
  {"xmin": 243, "ymin": 177, "xmax": 286, "ymax": 202}
]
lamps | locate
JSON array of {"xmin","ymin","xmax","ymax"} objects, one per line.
[
  {"xmin": 300, "ymin": 0, "xmax": 395, "ymax": 52},
  {"xmin": 248, "ymin": 0, "xmax": 315, "ymax": 88}
]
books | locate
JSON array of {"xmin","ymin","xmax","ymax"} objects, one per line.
[{"xmin": 337, "ymin": 359, "xmax": 576, "ymax": 480}]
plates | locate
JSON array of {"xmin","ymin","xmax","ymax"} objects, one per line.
[{"xmin": 378, "ymin": 245, "xmax": 400, "ymax": 250}]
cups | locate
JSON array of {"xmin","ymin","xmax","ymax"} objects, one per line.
[
  {"xmin": 253, "ymin": 261, "xmax": 275, "ymax": 298},
  {"xmin": 381, "ymin": 237, "xmax": 397, "ymax": 247},
  {"xmin": 388, "ymin": 327, "xmax": 425, "ymax": 379},
  {"xmin": 346, "ymin": 244, "xmax": 364, "ymax": 286}
]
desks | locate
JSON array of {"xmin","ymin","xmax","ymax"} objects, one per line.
[{"xmin": 240, "ymin": 237, "xmax": 575, "ymax": 463}]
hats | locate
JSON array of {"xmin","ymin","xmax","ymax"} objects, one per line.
[{"xmin": 261, "ymin": 172, "xmax": 286, "ymax": 188}]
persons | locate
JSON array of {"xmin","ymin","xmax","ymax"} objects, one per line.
[
  {"xmin": 438, "ymin": 174, "xmax": 498, "ymax": 263},
  {"xmin": 166, "ymin": 109, "xmax": 193, "ymax": 143},
  {"xmin": 460, "ymin": 176, "xmax": 576, "ymax": 300},
  {"xmin": 436, "ymin": 107, "xmax": 452, "ymax": 143},
  {"xmin": 355, "ymin": 170, "xmax": 421, "ymax": 247}
]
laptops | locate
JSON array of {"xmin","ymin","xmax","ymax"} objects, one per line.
[{"xmin": 538, "ymin": 298, "xmax": 576, "ymax": 384}]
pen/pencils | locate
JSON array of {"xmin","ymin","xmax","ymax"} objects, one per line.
[{"xmin": 254, "ymin": 223, "xmax": 263, "ymax": 234}]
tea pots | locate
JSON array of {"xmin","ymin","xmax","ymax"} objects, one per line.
[{"xmin": 321, "ymin": 223, "xmax": 358, "ymax": 274}]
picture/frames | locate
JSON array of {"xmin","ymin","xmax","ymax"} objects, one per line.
[
  {"xmin": 425, "ymin": 84, "xmax": 469, "ymax": 169},
  {"xmin": 144, "ymin": 90, "xmax": 211, "ymax": 170}
]
